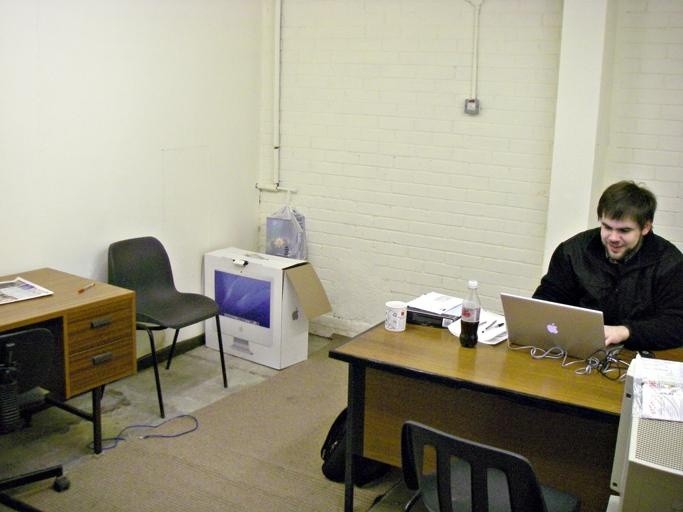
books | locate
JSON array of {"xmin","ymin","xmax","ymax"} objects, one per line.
[{"xmin": 405, "ymin": 306, "xmax": 458, "ymax": 329}]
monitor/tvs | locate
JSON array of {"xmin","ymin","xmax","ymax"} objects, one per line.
[{"xmin": 213, "ymin": 270, "xmax": 275, "ymax": 355}]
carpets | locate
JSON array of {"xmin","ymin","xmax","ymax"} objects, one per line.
[
  {"xmin": 370, "ymin": 481, "xmax": 428, "ymax": 512},
  {"xmin": 0, "ymin": 334, "xmax": 400, "ymax": 512}
]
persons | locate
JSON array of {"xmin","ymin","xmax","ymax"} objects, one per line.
[{"xmin": 529, "ymin": 178, "xmax": 682, "ymax": 351}]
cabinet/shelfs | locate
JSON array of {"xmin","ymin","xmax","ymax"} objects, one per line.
[{"xmin": 599, "ymin": 354, "xmax": 683, "ymax": 512}]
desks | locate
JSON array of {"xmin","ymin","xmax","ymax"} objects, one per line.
[
  {"xmin": 326, "ymin": 319, "xmax": 683, "ymax": 512},
  {"xmin": 0, "ymin": 265, "xmax": 138, "ymax": 456}
]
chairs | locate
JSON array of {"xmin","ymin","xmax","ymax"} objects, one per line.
[
  {"xmin": 394, "ymin": 412, "xmax": 580, "ymax": 512},
  {"xmin": 96, "ymin": 233, "xmax": 231, "ymax": 420},
  {"xmin": 0, "ymin": 326, "xmax": 81, "ymax": 510}
]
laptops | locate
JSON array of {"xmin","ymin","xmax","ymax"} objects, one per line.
[{"xmin": 500, "ymin": 291, "xmax": 625, "ymax": 365}]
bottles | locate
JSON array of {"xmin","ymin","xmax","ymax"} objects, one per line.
[{"xmin": 457, "ymin": 279, "xmax": 482, "ymax": 348}]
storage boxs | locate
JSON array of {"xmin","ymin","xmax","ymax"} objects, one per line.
[{"xmin": 201, "ymin": 245, "xmax": 332, "ymax": 372}]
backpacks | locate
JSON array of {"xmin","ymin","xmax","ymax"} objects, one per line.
[{"xmin": 319, "ymin": 406, "xmax": 391, "ymax": 486}]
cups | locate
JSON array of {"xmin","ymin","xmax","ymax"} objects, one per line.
[{"xmin": 384, "ymin": 301, "xmax": 406, "ymax": 332}]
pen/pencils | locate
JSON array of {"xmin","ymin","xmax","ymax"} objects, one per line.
[
  {"xmin": 479, "ymin": 320, "xmax": 504, "ymax": 333},
  {"xmin": 78, "ymin": 282, "xmax": 96, "ymax": 292}
]
